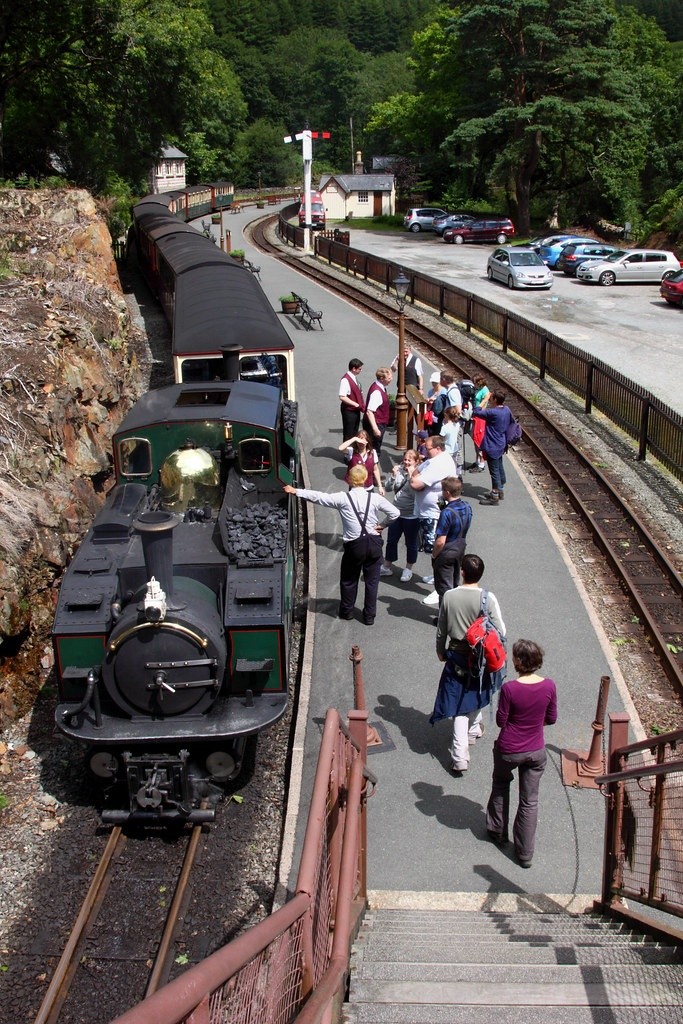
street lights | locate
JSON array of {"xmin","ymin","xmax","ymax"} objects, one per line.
[
  {"xmin": 257, "ymin": 171, "xmax": 262, "ymax": 201},
  {"xmin": 391, "ymin": 267, "xmax": 414, "ymax": 451},
  {"xmin": 217, "ymin": 195, "xmax": 225, "ymax": 250}
]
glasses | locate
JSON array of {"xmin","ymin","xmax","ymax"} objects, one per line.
[{"xmin": 425, "ymin": 445, "xmax": 437, "ymax": 452}]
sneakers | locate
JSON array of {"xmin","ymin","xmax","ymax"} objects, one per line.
[
  {"xmin": 400, "ymin": 568, "xmax": 413, "ymax": 581},
  {"xmin": 379, "ymin": 565, "xmax": 393, "ymax": 576},
  {"xmin": 422, "ymin": 590, "xmax": 441, "ymax": 605},
  {"xmin": 481, "ymin": 495, "xmax": 500, "ymax": 506},
  {"xmin": 422, "ymin": 575, "xmax": 436, "ymax": 584},
  {"xmin": 485, "ymin": 491, "xmax": 504, "ymax": 500}
]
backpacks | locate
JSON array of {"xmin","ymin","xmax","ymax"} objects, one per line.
[{"xmin": 432, "ymin": 387, "xmax": 459, "ymax": 419}]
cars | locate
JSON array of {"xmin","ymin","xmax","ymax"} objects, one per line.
[
  {"xmin": 555, "ymin": 242, "xmax": 620, "ymax": 277},
  {"xmin": 432, "ymin": 213, "xmax": 476, "ymax": 235},
  {"xmin": 660, "ymin": 268, "xmax": 683, "ymax": 309},
  {"xmin": 514, "ymin": 235, "xmax": 600, "ymax": 267},
  {"xmin": 486, "ymin": 247, "xmax": 553, "ymax": 289}
]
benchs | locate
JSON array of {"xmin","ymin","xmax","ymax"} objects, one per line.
[
  {"xmin": 292, "ymin": 291, "xmax": 322, "ymax": 331},
  {"xmin": 201, "ymin": 220, "xmax": 212, "ymax": 231},
  {"xmin": 243, "ymin": 259, "xmax": 261, "ymax": 281},
  {"xmin": 207, "ymin": 231, "xmax": 216, "ymax": 244},
  {"xmin": 229, "ymin": 201, "xmax": 243, "ymax": 214},
  {"xmin": 268, "ymin": 196, "xmax": 281, "ymax": 205}
]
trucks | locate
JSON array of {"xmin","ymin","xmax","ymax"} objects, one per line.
[{"xmin": 299, "ymin": 190, "xmax": 325, "ymax": 228}]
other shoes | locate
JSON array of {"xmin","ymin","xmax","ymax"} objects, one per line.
[
  {"xmin": 449, "ymin": 761, "xmax": 467, "ymax": 771},
  {"xmin": 363, "ymin": 614, "xmax": 374, "ymax": 625},
  {"xmin": 466, "ymin": 462, "xmax": 485, "ymax": 473},
  {"xmin": 515, "ymin": 855, "xmax": 532, "ymax": 868},
  {"xmin": 488, "ymin": 829, "xmax": 510, "ymax": 842},
  {"xmin": 469, "ymin": 723, "xmax": 485, "ymax": 744},
  {"xmin": 337, "ymin": 607, "xmax": 354, "ymax": 619},
  {"xmin": 389, "ymin": 423, "xmax": 398, "ymax": 435}
]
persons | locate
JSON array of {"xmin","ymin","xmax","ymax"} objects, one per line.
[
  {"xmin": 407, "ymin": 436, "xmax": 456, "ymax": 604},
  {"xmin": 472, "ymin": 389, "xmax": 512, "ymax": 506},
  {"xmin": 337, "ymin": 358, "xmax": 365, "ymax": 463},
  {"xmin": 411, "ymin": 427, "xmax": 431, "ymax": 460},
  {"xmin": 283, "ymin": 463, "xmax": 400, "ymax": 626},
  {"xmin": 429, "ymin": 477, "xmax": 473, "ymax": 626},
  {"xmin": 378, "ymin": 449, "xmax": 421, "ymax": 579},
  {"xmin": 391, "ymin": 344, "xmax": 424, "ymax": 437},
  {"xmin": 362, "ymin": 367, "xmax": 392, "ymax": 457},
  {"xmin": 486, "ymin": 639, "xmax": 558, "ymax": 869},
  {"xmin": 427, "ymin": 559, "xmax": 505, "ymax": 773},
  {"xmin": 424, "ymin": 369, "xmax": 491, "ymax": 495}
]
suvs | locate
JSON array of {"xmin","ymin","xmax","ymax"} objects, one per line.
[
  {"xmin": 441, "ymin": 215, "xmax": 514, "ymax": 246},
  {"xmin": 403, "ymin": 208, "xmax": 448, "ymax": 232},
  {"xmin": 575, "ymin": 248, "xmax": 683, "ymax": 286}
]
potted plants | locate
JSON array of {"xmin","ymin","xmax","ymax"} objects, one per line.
[
  {"xmin": 279, "ymin": 296, "xmax": 300, "ymax": 314},
  {"xmin": 256, "ymin": 201, "xmax": 264, "ymax": 208},
  {"xmin": 387, "ymin": 394, "xmax": 397, "ymax": 425},
  {"xmin": 212, "ymin": 214, "xmax": 222, "ymax": 224},
  {"xmin": 228, "ymin": 249, "xmax": 245, "ymax": 261}
]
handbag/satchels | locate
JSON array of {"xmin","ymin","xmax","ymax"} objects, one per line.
[
  {"xmin": 504, "ymin": 405, "xmax": 523, "ymax": 445},
  {"xmin": 464, "ymin": 590, "xmax": 508, "ymax": 673}
]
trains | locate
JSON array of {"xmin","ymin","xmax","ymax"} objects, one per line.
[{"xmin": 48, "ymin": 176, "xmax": 300, "ymax": 831}]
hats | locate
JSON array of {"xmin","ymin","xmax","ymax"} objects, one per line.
[
  {"xmin": 430, "ymin": 371, "xmax": 442, "ymax": 383},
  {"xmin": 413, "ymin": 429, "xmax": 428, "ymax": 439}
]
